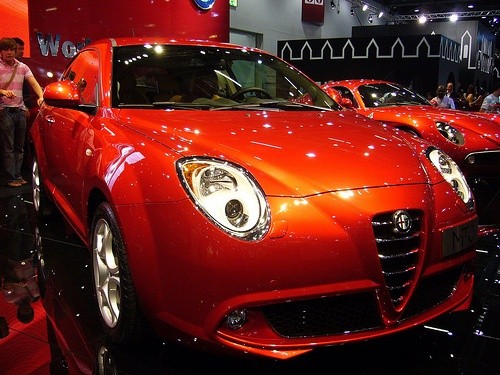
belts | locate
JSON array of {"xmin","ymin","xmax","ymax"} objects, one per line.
[{"xmin": 7, "ymin": 107, "xmax": 20, "ymax": 112}]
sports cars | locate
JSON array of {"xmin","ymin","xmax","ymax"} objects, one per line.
[
  {"xmin": 26, "ymin": 37, "xmax": 479, "ymax": 366},
  {"xmin": 285, "ymin": 80, "xmax": 500, "ymax": 246}
]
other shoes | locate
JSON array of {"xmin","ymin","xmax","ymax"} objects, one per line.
[{"xmin": 6, "ymin": 180, "xmax": 28, "ymax": 187}]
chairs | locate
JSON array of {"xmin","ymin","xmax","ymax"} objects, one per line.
[{"xmin": 187, "ymin": 70, "xmax": 233, "ymax": 102}]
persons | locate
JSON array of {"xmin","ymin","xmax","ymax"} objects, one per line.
[
  {"xmin": 0, "ymin": 37, "xmax": 44, "ymax": 186},
  {"xmin": 430, "ymin": 82, "xmax": 500, "ymax": 113}
]
